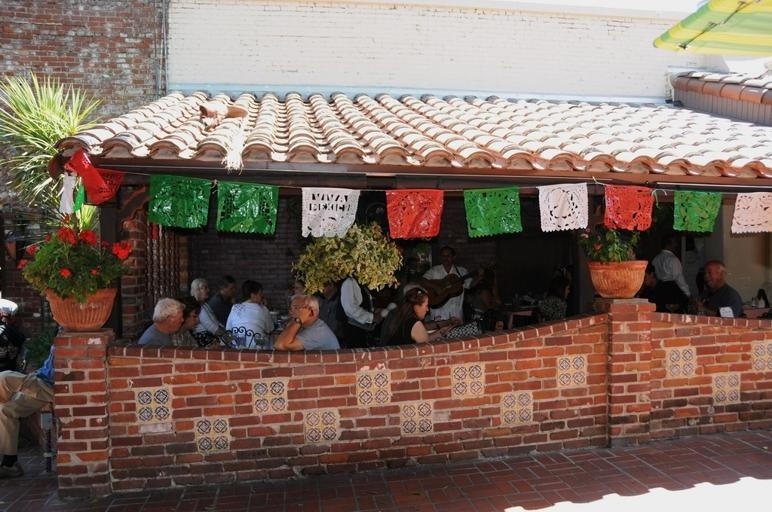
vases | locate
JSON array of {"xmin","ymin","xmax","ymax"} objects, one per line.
[
  {"xmin": 44, "ymin": 288, "xmax": 118, "ymax": 332},
  {"xmin": 587, "ymin": 259, "xmax": 649, "ymax": 299}
]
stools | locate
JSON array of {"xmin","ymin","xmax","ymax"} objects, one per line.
[{"xmin": 40, "ymin": 403, "xmax": 56, "ymax": 474}]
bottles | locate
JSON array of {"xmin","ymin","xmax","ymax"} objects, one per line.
[{"xmin": 751, "ymin": 296, "xmax": 756, "ymax": 307}]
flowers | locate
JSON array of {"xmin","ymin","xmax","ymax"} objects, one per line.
[
  {"xmin": 575, "ymin": 223, "xmax": 641, "ymax": 262},
  {"xmin": 15, "ymin": 213, "xmax": 135, "ymax": 305}
]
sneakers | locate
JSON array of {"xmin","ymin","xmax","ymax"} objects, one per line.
[{"xmin": 1, "ymin": 461, "xmax": 24, "ymax": 478}]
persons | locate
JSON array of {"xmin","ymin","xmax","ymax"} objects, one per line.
[
  {"xmin": 752, "ymin": 289, "xmax": 770, "ymax": 308},
  {"xmin": 694, "ymin": 260, "xmax": 743, "ymax": 316},
  {"xmin": 0, "ymin": 328, "xmax": 56, "ymax": 476},
  {"xmin": 274, "ymin": 274, "xmax": 382, "ymax": 351},
  {"xmin": 137, "ymin": 275, "xmax": 237, "ymax": 351},
  {"xmin": 381, "ymin": 246, "xmax": 570, "ymax": 345},
  {"xmin": 651, "ymin": 249, "xmax": 689, "ymax": 314},
  {"xmin": 225, "ymin": 279, "xmax": 276, "ymax": 351},
  {"xmin": 635, "ymin": 268, "xmax": 658, "ymax": 298}
]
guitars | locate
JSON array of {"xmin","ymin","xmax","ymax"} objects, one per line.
[{"xmin": 416, "ymin": 268, "xmax": 484, "ymax": 309}]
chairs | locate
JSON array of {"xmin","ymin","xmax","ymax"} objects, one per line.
[{"xmin": 219, "ymin": 325, "xmax": 262, "ymax": 349}]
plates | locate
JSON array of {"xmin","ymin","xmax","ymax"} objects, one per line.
[{"xmin": 506, "ymin": 301, "xmax": 537, "ymax": 309}]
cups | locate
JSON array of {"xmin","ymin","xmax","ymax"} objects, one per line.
[{"xmin": 665, "ymin": 303, "xmax": 681, "ymax": 312}]
